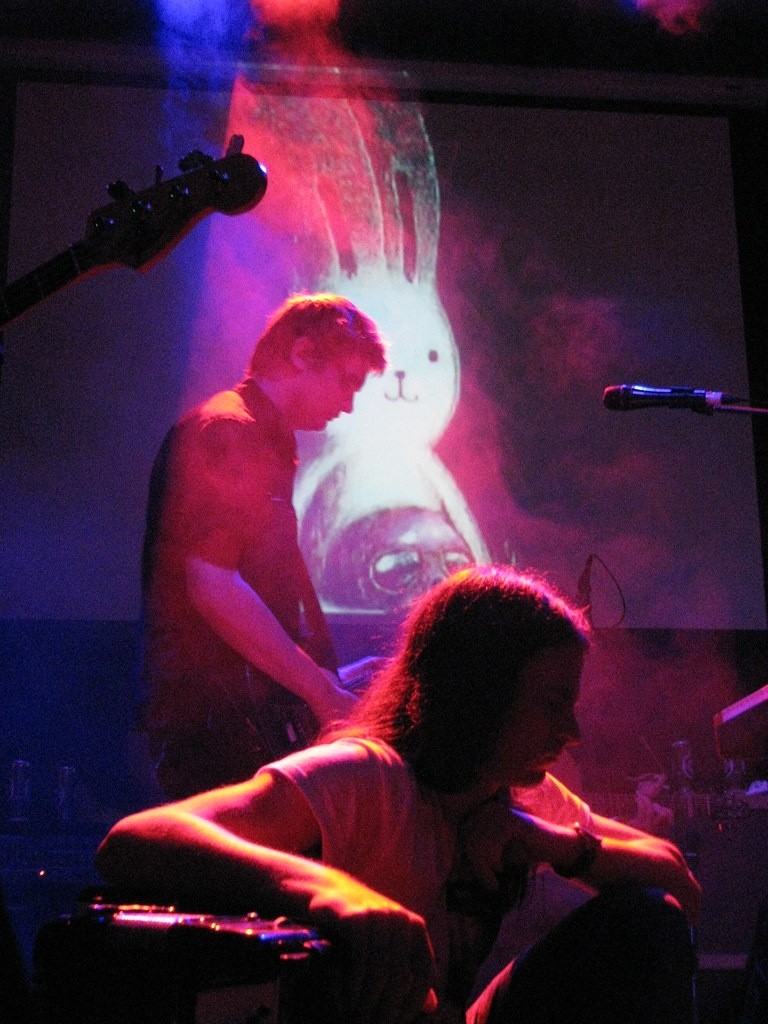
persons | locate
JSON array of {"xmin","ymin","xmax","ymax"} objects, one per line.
[
  {"xmin": 94, "ymin": 569, "xmax": 704, "ymax": 1024},
  {"xmin": 142, "ymin": 292, "xmax": 386, "ymax": 809}
]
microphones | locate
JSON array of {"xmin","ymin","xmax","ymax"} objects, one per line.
[
  {"xmin": 573, "ymin": 555, "xmax": 593, "ymax": 627},
  {"xmin": 602, "ymin": 383, "xmax": 740, "ymax": 411}
]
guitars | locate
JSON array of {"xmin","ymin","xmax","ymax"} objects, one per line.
[{"xmin": 0, "ymin": 132, "xmax": 268, "ymax": 330}]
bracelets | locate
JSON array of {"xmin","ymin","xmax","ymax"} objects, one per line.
[{"xmin": 552, "ymin": 823, "xmax": 602, "ymax": 879}]
